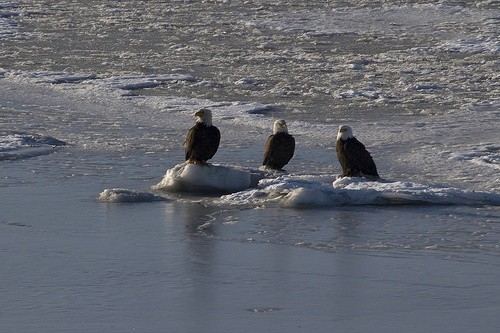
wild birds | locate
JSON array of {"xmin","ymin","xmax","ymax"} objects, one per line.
[
  {"xmin": 334, "ymin": 123, "xmax": 382, "ymax": 183},
  {"xmin": 263, "ymin": 118, "xmax": 296, "ymax": 170},
  {"xmin": 185, "ymin": 108, "xmax": 220, "ymax": 168}
]
